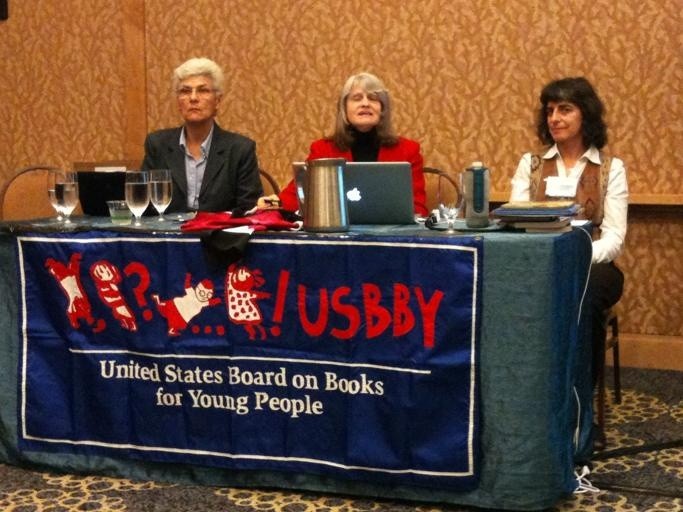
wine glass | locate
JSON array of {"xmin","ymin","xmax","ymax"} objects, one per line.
[
  {"xmin": 124, "ymin": 169, "xmax": 151, "ymax": 228},
  {"xmin": 46, "ymin": 169, "xmax": 68, "ymax": 228},
  {"xmin": 146, "ymin": 168, "xmax": 174, "ymax": 223},
  {"xmin": 435, "ymin": 170, "xmax": 469, "ymax": 237},
  {"xmin": 53, "ymin": 170, "xmax": 80, "ymax": 230}
]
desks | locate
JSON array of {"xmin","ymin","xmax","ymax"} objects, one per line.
[{"xmin": 2, "ymin": 202, "xmax": 594, "ymax": 501}]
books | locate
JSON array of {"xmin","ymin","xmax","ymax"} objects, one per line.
[{"xmin": 491, "ymin": 200, "xmax": 584, "ymax": 235}]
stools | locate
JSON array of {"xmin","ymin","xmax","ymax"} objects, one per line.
[{"xmin": 594, "ymin": 305, "xmax": 622, "ymax": 424}]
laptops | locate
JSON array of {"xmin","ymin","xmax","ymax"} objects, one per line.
[{"xmin": 292, "ymin": 161, "xmax": 414, "ymax": 225}]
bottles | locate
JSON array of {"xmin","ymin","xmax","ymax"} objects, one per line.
[{"xmin": 461, "ymin": 160, "xmax": 490, "ymax": 230}]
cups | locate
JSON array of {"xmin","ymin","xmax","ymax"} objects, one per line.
[{"xmin": 105, "ymin": 199, "xmax": 134, "ymax": 227}]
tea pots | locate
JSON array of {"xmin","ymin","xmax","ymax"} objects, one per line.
[{"xmin": 291, "ymin": 156, "xmax": 351, "ymax": 234}]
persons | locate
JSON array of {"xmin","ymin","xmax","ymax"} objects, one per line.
[
  {"xmin": 132, "ymin": 57, "xmax": 268, "ymax": 216},
  {"xmin": 274, "ymin": 72, "xmax": 432, "ymax": 221},
  {"xmin": 508, "ymin": 76, "xmax": 630, "ymax": 474}
]
